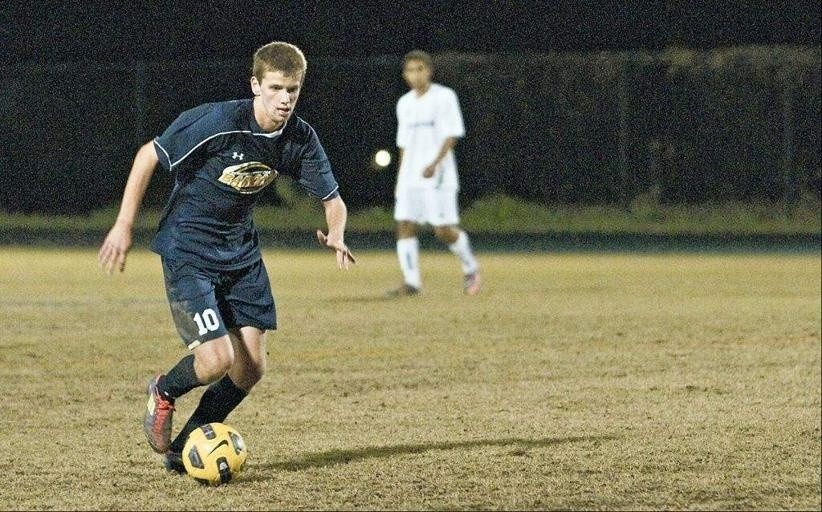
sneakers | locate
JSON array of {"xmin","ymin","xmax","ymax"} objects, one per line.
[{"xmin": 384, "ymin": 272, "xmax": 484, "ymax": 300}]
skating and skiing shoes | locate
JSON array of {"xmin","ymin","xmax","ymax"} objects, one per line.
[{"xmin": 142, "ymin": 374, "xmax": 185, "ymax": 472}]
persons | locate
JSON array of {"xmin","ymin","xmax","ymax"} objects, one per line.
[
  {"xmin": 382, "ymin": 49, "xmax": 482, "ymax": 302},
  {"xmin": 96, "ymin": 39, "xmax": 360, "ymax": 476}
]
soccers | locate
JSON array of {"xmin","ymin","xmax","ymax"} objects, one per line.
[{"xmin": 181, "ymin": 422, "xmax": 247, "ymax": 487}]
